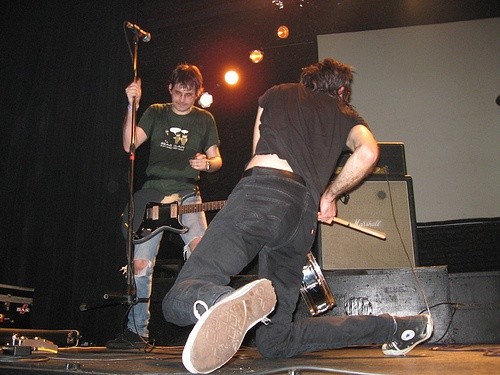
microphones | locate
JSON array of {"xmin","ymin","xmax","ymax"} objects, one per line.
[{"xmin": 125, "ymin": 21, "xmax": 151, "ymax": 42}]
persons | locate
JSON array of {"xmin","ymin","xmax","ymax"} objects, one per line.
[
  {"xmin": 162, "ymin": 58, "xmax": 434, "ymax": 375},
  {"xmin": 106, "ymin": 64, "xmax": 222, "ymax": 349}
]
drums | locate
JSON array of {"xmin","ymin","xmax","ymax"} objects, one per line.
[{"xmin": 300, "ymin": 252, "xmax": 335, "ymax": 316}]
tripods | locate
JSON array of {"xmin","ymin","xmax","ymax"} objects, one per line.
[{"xmin": 79, "ymin": 31, "xmax": 165, "ymax": 311}]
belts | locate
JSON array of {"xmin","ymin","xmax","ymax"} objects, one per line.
[{"xmin": 241, "ymin": 166, "xmax": 306, "ymax": 187}]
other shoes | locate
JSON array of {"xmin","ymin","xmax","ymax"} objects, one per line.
[{"xmin": 104, "ymin": 328, "xmax": 148, "ymax": 349}]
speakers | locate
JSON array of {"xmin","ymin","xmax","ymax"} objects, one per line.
[{"xmin": 315, "ymin": 175, "xmax": 418, "ymax": 270}]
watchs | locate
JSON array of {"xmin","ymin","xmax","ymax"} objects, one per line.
[{"xmin": 207, "ymin": 160, "xmax": 211, "ymax": 172}]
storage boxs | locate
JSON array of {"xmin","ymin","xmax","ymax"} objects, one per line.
[{"xmin": 0, "ymin": 284, "xmax": 35, "ymax": 345}]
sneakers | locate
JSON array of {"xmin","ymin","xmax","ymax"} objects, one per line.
[
  {"xmin": 381, "ymin": 313, "xmax": 433, "ymax": 357},
  {"xmin": 181, "ymin": 277, "xmax": 277, "ymax": 375}
]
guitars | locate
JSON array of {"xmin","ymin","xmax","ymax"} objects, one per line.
[{"xmin": 120, "ymin": 187, "xmax": 227, "ymax": 244}]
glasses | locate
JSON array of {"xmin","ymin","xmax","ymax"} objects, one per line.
[{"xmin": 171, "ymin": 89, "xmax": 196, "ymax": 100}]
